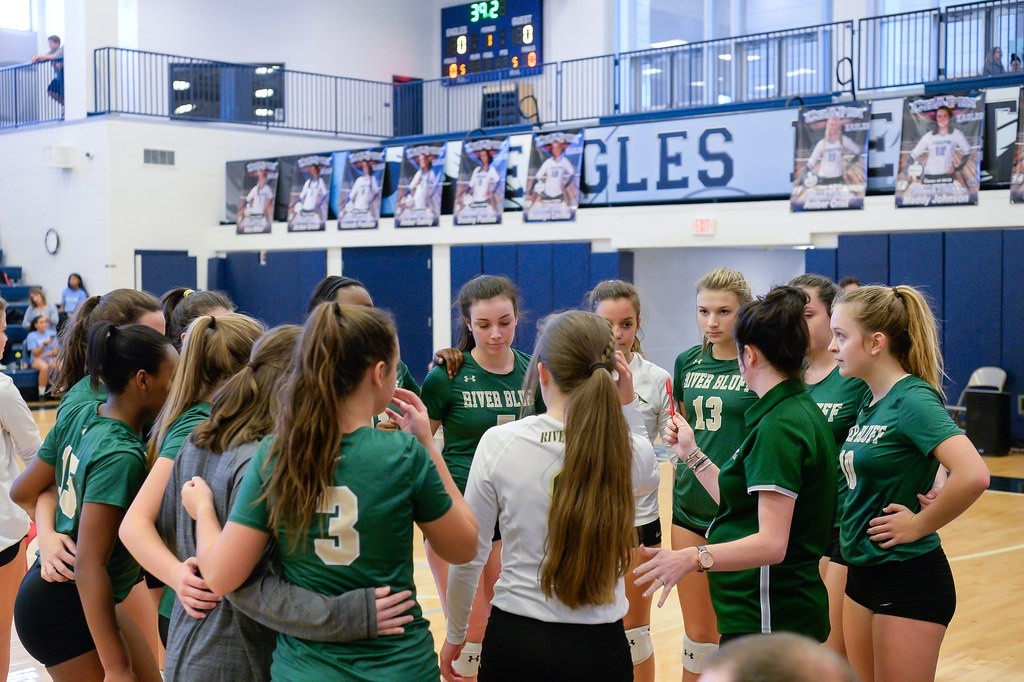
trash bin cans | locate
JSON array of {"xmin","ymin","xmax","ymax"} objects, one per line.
[{"xmin": 966, "ymin": 390, "xmax": 1012, "ymax": 457}]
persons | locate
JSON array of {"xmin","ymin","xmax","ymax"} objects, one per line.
[
  {"xmin": 904, "ymin": 105, "xmax": 971, "ymax": 179},
  {"xmin": 155, "ymin": 326, "xmax": 414, "ymax": 682},
  {"xmin": 633, "ymin": 285, "xmax": 837, "ymax": 681},
  {"xmin": 236, "ymin": 140, "xmax": 576, "ymax": 228},
  {"xmin": 22, "ymin": 274, "xmax": 90, "ymax": 403},
  {"xmin": 798, "ymin": 113, "xmax": 862, "ymax": 184},
  {"xmin": 984, "ymin": 47, "xmax": 1006, "ymax": 74},
  {"xmin": 33, "ymin": 35, "xmax": 64, "ymax": 105},
  {"xmin": 179, "ymin": 303, "xmax": 478, "ymax": 682},
  {"xmin": 697, "ymin": 631, "xmax": 856, "ymax": 682},
  {"xmin": 828, "ymin": 285, "xmax": 991, "ymax": 682},
  {"xmin": 440, "ymin": 311, "xmax": 660, "ymax": 682},
  {"xmin": 307, "ymin": 276, "xmax": 464, "ymax": 429},
  {"xmin": 789, "ymin": 274, "xmax": 948, "ymax": 663},
  {"xmin": 420, "ymin": 276, "xmax": 548, "ymax": 682},
  {"xmin": 588, "ymin": 281, "xmax": 678, "ymax": 682},
  {"xmin": 0, "ymin": 287, "xmax": 265, "ymax": 681},
  {"xmin": 671, "ymin": 267, "xmax": 760, "ymax": 682},
  {"xmin": 1009, "ymin": 53, "xmax": 1021, "ymax": 72}
]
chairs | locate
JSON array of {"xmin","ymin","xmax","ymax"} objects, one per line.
[{"xmin": 946, "ymin": 365, "xmax": 1007, "ymax": 433}]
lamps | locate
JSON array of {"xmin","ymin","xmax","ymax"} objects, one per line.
[{"xmin": 36, "ymin": 147, "xmax": 75, "ymax": 169}]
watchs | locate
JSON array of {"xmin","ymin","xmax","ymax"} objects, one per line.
[{"xmin": 697, "ymin": 546, "xmax": 714, "ymax": 572}]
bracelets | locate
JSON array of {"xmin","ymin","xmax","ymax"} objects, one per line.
[
  {"xmin": 684, "ymin": 448, "xmax": 712, "ymax": 474},
  {"xmin": 44, "ymin": 343, "xmax": 48, "ymax": 346}
]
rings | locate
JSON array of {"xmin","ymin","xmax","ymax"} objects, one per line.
[
  {"xmin": 658, "ymin": 576, "xmax": 665, "ymax": 585},
  {"xmin": 679, "ymin": 424, "xmax": 685, "ymax": 428}
]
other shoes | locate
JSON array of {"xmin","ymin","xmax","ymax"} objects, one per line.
[{"xmin": 39, "ymin": 394, "xmax": 46, "ymax": 401}]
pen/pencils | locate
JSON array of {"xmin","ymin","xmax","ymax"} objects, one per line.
[{"xmin": 666, "ymin": 377, "xmax": 674, "ymax": 418}]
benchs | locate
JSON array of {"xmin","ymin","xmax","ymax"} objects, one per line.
[{"xmin": 0, "ymin": 249, "xmax": 64, "ymax": 388}]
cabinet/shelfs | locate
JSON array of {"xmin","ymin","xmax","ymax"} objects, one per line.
[{"xmin": 481, "ymin": 82, "xmax": 534, "ymax": 126}]
